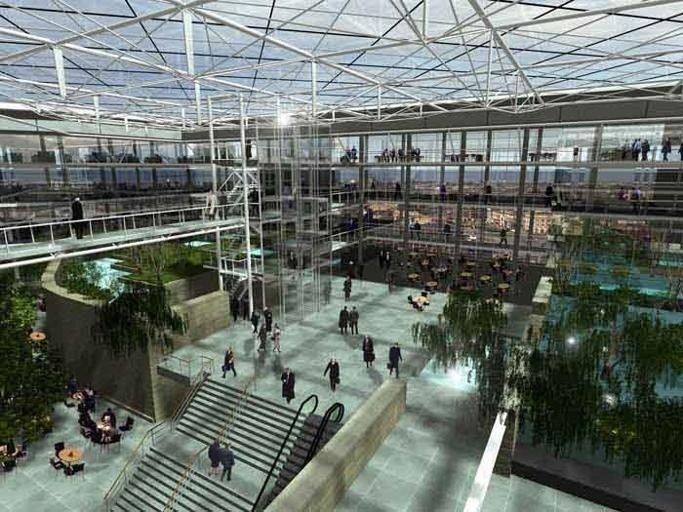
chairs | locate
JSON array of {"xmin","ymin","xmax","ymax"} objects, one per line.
[{"xmin": 0, "ymin": 386, "xmax": 136, "ymax": 483}]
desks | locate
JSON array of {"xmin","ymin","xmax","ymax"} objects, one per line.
[{"xmin": 333, "ymin": 149, "xmax": 519, "ymax": 310}]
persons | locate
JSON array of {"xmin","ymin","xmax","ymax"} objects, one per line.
[
  {"xmin": 64, "ymin": 376, "xmax": 114, "ymax": 427},
  {"xmin": 251, "ymin": 187, "xmax": 259, "ymax": 215},
  {"xmin": 71, "ymin": 197, "xmax": 83, "ymax": 239},
  {"xmin": 206, "ymin": 189, "xmax": 218, "ymax": 221},
  {"xmin": 217, "ymin": 190, "xmax": 227, "ymax": 220},
  {"xmin": 323, "ymin": 357, "xmax": 339, "ymax": 392},
  {"xmin": 221, "ymin": 346, "xmax": 236, "ymax": 379},
  {"xmin": 362, "ymin": 334, "xmax": 375, "ymax": 368},
  {"xmin": 349, "ymin": 306, "xmax": 359, "ymax": 335},
  {"xmin": 340, "ymin": 306, "xmax": 350, "ymax": 334},
  {"xmin": 220, "ymin": 443, "xmax": 235, "ymax": 482},
  {"xmin": 342, "ymin": 137, "xmax": 683, "ymax": 303},
  {"xmin": 208, "ymin": 437, "xmax": 221, "ymax": 477},
  {"xmin": 281, "ymin": 368, "xmax": 295, "ymax": 404},
  {"xmin": 233, "ymin": 294, "xmax": 281, "ymax": 351},
  {"xmin": 389, "ymin": 342, "xmax": 403, "ymax": 379}
]
